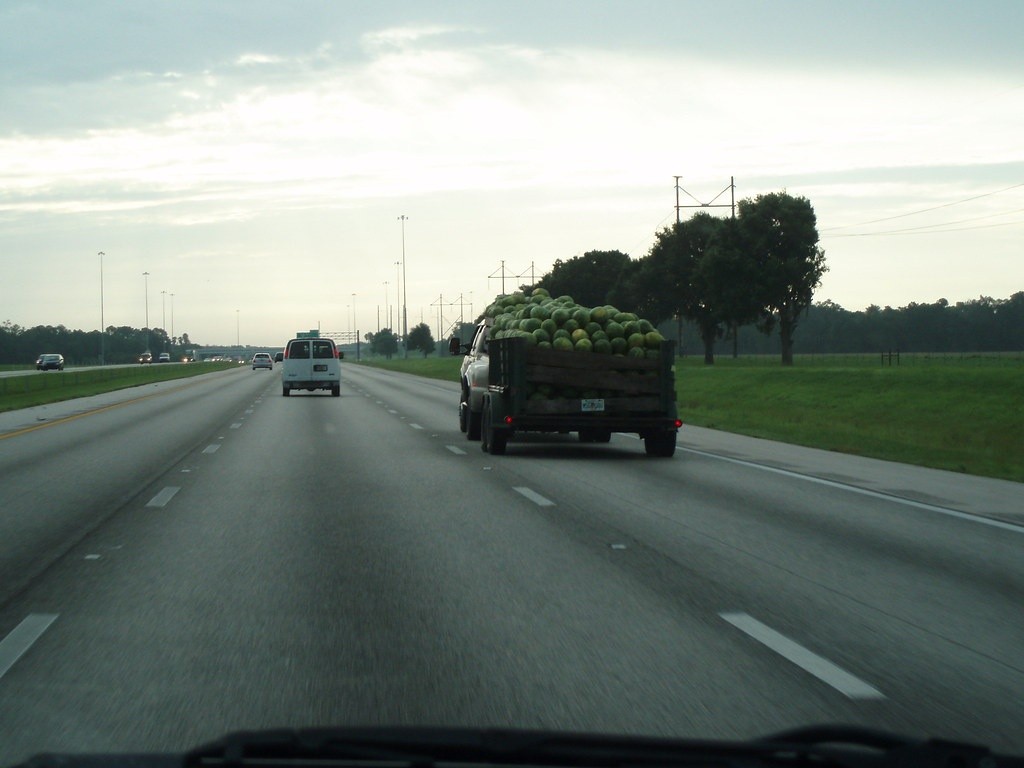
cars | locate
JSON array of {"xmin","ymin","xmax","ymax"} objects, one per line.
[
  {"xmin": 274, "ymin": 351, "xmax": 284, "ymax": 363},
  {"xmin": 36, "ymin": 353, "xmax": 64, "ymax": 371},
  {"xmin": 159, "ymin": 352, "xmax": 171, "ymax": 363},
  {"xmin": 140, "ymin": 352, "xmax": 152, "ymax": 364},
  {"xmin": 183, "ymin": 354, "xmax": 245, "ymax": 363},
  {"xmin": 251, "ymin": 353, "xmax": 274, "ymax": 371}
]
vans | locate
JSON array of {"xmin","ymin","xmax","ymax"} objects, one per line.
[{"xmin": 280, "ymin": 337, "xmax": 344, "ymax": 397}]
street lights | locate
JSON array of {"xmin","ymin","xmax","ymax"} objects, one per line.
[
  {"xmin": 170, "ymin": 293, "xmax": 175, "ymax": 340},
  {"xmin": 161, "ymin": 291, "xmax": 167, "ymax": 331},
  {"xmin": 398, "ymin": 214, "xmax": 410, "ymax": 358},
  {"xmin": 142, "ymin": 271, "xmax": 149, "ymax": 352},
  {"xmin": 394, "ymin": 261, "xmax": 403, "ymax": 335},
  {"xmin": 97, "ymin": 251, "xmax": 105, "ymax": 366},
  {"xmin": 383, "ymin": 281, "xmax": 390, "ymax": 328}
]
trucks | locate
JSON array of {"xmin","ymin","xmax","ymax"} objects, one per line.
[{"xmin": 449, "ymin": 316, "xmax": 683, "ymax": 457}]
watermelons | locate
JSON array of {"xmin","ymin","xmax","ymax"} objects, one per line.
[{"xmin": 486, "ymin": 288, "xmax": 667, "ymax": 359}]
